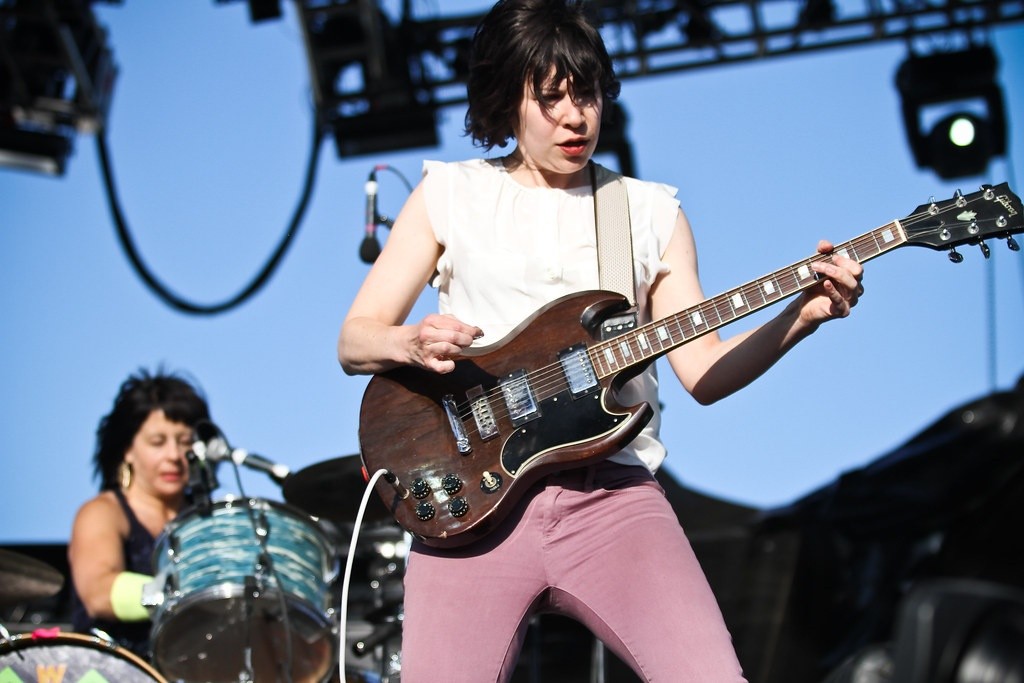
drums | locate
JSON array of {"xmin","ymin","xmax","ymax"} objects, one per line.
[{"xmin": 1, "ymin": 497, "xmax": 336, "ymax": 683}]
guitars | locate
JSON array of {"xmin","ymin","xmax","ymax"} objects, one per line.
[{"xmin": 359, "ymin": 183, "xmax": 1024, "ymax": 549}]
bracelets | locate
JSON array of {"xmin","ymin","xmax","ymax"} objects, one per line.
[{"xmin": 110, "ymin": 570, "xmax": 157, "ymax": 622}]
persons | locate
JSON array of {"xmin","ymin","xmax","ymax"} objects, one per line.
[
  {"xmin": 42, "ymin": 360, "xmax": 215, "ymax": 665},
  {"xmin": 337, "ymin": 0, "xmax": 867, "ymax": 683}
]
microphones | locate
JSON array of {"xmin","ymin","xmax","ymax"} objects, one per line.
[
  {"xmin": 359, "ymin": 168, "xmax": 381, "ymax": 263},
  {"xmin": 206, "ymin": 438, "xmax": 290, "ymax": 480}
]
